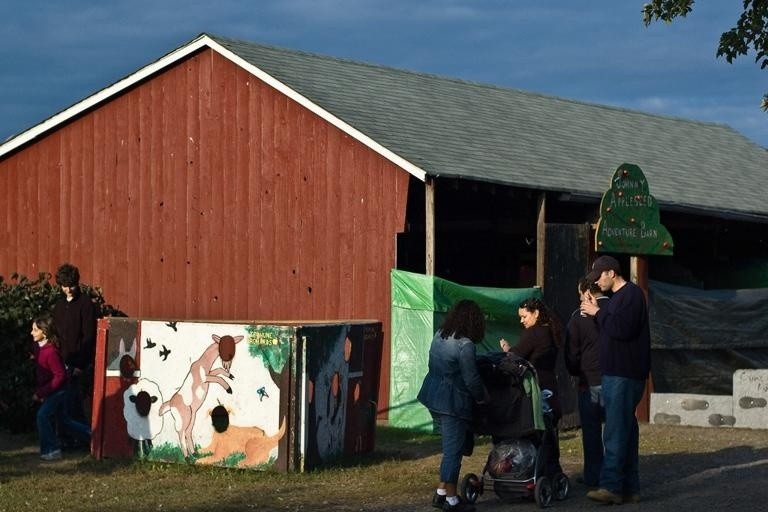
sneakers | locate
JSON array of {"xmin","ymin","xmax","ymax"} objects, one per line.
[
  {"xmin": 40, "ymin": 448, "xmax": 62, "ymax": 460},
  {"xmin": 432, "ymin": 489, "xmax": 476, "ymax": 512},
  {"xmin": 576, "ymin": 478, "xmax": 640, "ymax": 505}
]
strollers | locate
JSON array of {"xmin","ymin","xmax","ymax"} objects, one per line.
[{"xmin": 461, "ymin": 353, "xmax": 570, "ymax": 508}]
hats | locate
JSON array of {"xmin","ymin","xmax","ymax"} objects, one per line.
[{"xmin": 585, "ymin": 256, "xmax": 619, "ymax": 282}]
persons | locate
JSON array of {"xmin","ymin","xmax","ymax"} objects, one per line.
[
  {"xmin": 29, "ymin": 315, "xmax": 92, "ymax": 460},
  {"xmin": 580, "ymin": 256, "xmax": 649, "ymax": 506},
  {"xmin": 500, "ymin": 298, "xmax": 566, "ymax": 477},
  {"xmin": 52, "ymin": 263, "xmax": 98, "ymax": 451},
  {"xmin": 417, "ymin": 300, "xmax": 492, "ymax": 512},
  {"xmin": 564, "ymin": 272, "xmax": 611, "ymax": 488}
]
